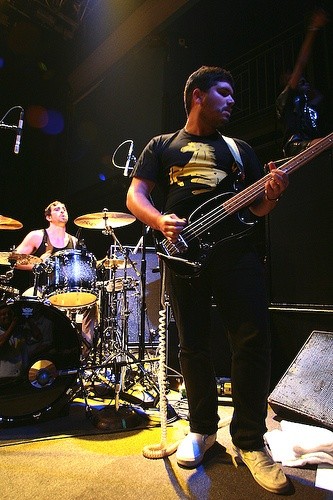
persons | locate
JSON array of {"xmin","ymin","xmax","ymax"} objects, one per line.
[
  {"xmin": 11, "ymin": 201, "xmax": 109, "ymax": 284},
  {"xmin": 125, "ymin": 67, "xmax": 289, "ymax": 494}
]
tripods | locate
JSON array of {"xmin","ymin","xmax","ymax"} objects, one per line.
[{"xmin": 123, "ymin": 150, "xmax": 160, "ymax": 402}]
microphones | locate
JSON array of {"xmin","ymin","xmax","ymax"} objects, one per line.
[
  {"xmin": 13, "ymin": 109, "xmax": 25, "ymax": 154},
  {"xmin": 123, "ymin": 141, "xmax": 134, "ymax": 177}
]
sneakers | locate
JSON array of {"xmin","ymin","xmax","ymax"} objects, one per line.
[
  {"xmin": 174, "ymin": 430, "xmax": 220, "ymax": 467},
  {"xmin": 230, "ymin": 438, "xmax": 292, "ymax": 495}
]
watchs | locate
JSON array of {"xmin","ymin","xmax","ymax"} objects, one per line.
[{"xmin": 268, "ymin": 194, "xmax": 282, "ymax": 203}]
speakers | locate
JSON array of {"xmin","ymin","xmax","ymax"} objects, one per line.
[{"xmin": 269, "ymin": 330, "xmax": 333, "ymax": 431}]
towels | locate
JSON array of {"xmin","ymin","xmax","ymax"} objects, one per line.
[{"xmin": 264, "ymin": 419, "xmax": 333, "ymax": 468}]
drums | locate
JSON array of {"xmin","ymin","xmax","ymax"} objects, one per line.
[
  {"xmin": 43, "ymin": 249, "xmax": 98, "ymax": 310},
  {"xmin": 1, "ymin": 296, "xmax": 82, "ymax": 423}
]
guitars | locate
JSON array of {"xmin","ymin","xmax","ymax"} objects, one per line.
[{"xmin": 155, "ymin": 134, "xmax": 333, "ymax": 276}]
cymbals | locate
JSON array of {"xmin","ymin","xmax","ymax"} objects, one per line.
[
  {"xmin": 96, "ymin": 259, "xmax": 133, "ymax": 269},
  {"xmin": 0, "ymin": 214, "xmax": 24, "ymax": 230},
  {"xmin": 0, "ymin": 252, "xmax": 42, "ymax": 266},
  {"xmin": 73, "ymin": 212, "xmax": 135, "ymax": 229},
  {"xmin": 106, "ymin": 277, "xmax": 135, "ymax": 292}
]
